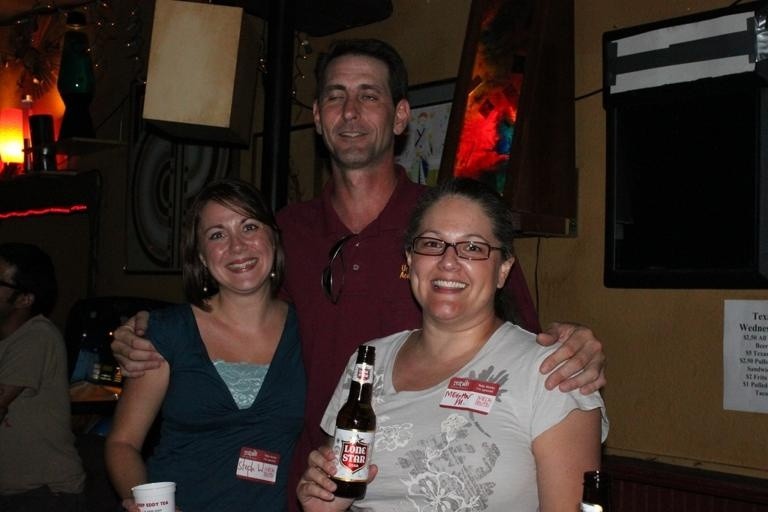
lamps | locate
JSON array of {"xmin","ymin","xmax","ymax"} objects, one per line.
[
  {"xmin": 291, "ymin": 32, "xmax": 314, "ymax": 108},
  {"xmin": 142, "ymin": 0, "xmax": 264, "ymax": 149}
]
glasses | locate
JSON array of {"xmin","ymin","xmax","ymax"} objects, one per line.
[
  {"xmin": 412, "ymin": 234, "xmax": 505, "ymax": 261},
  {"xmin": 320, "ymin": 233, "xmax": 358, "ymax": 305}
]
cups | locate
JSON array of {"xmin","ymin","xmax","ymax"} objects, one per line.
[{"xmin": 131, "ymin": 480, "xmax": 177, "ymax": 512}]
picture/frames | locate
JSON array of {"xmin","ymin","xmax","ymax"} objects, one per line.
[
  {"xmin": 393, "ymin": 75, "xmax": 458, "ymax": 185},
  {"xmin": 249, "ymin": 124, "xmax": 322, "ymax": 218},
  {"xmin": 124, "ymin": 79, "xmax": 246, "ymax": 276}
]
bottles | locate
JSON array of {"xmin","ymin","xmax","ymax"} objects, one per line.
[
  {"xmin": 81, "ymin": 325, "xmax": 125, "ymax": 386},
  {"xmin": 27, "ymin": 117, "xmax": 59, "ymax": 174},
  {"xmin": 329, "ymin": 345, "xmax": 377, "ymax": 500},
  {"xmin": 579, "ymin": 470, "xmax": 610, "ymax": 512},
  {"xmin": 55, "ymin": 29, "xmax": 98, "ymax": 139}
]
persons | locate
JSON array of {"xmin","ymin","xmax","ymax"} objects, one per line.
[
  {"xmin": 0, "ymin": 242, "xmax": 89, "ymax": 512},
  {"xmin": 409, "ymin": 113, "xmax": 432, "ymax": 185},
  {"xmin": 111, "ymin": 36, "xmax": 607, "ymax": 510},
  {"xmin": 296, "ymin": 174, "xmax": 611, "ymax": 510},
  {"xmin": 107, "ymin": 178, "xmax": 306, "ymax": 511}
]
